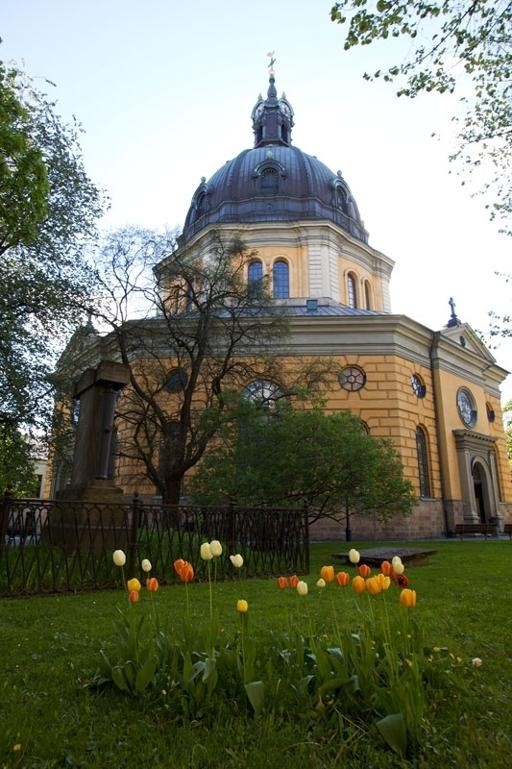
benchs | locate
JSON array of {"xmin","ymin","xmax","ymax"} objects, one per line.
[
  {"xmin": 454, "ymin": 524, "xmax": 497, "ymax": 540},
  {"xmin": 503, "ymin": 524, "xmax": 512, "ymax": 538}
]
